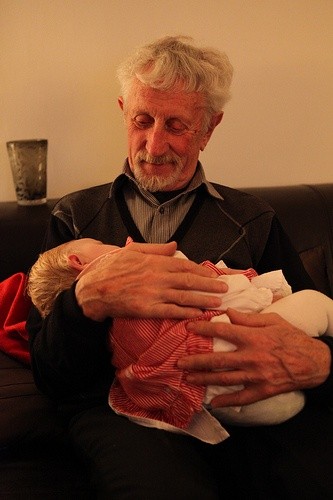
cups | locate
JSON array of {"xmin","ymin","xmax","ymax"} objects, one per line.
[{"xmin": 6, "ymin": 139, "xmax": 48, "ymax": 205}]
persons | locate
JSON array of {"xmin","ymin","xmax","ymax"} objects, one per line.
[
  {"xmin": 31, "ymin": 33, "xmax": 333, "ymax": 499},
  {"xmin": 28, "ymin": 237, "xmax": 333, "ymax": 445}
]
